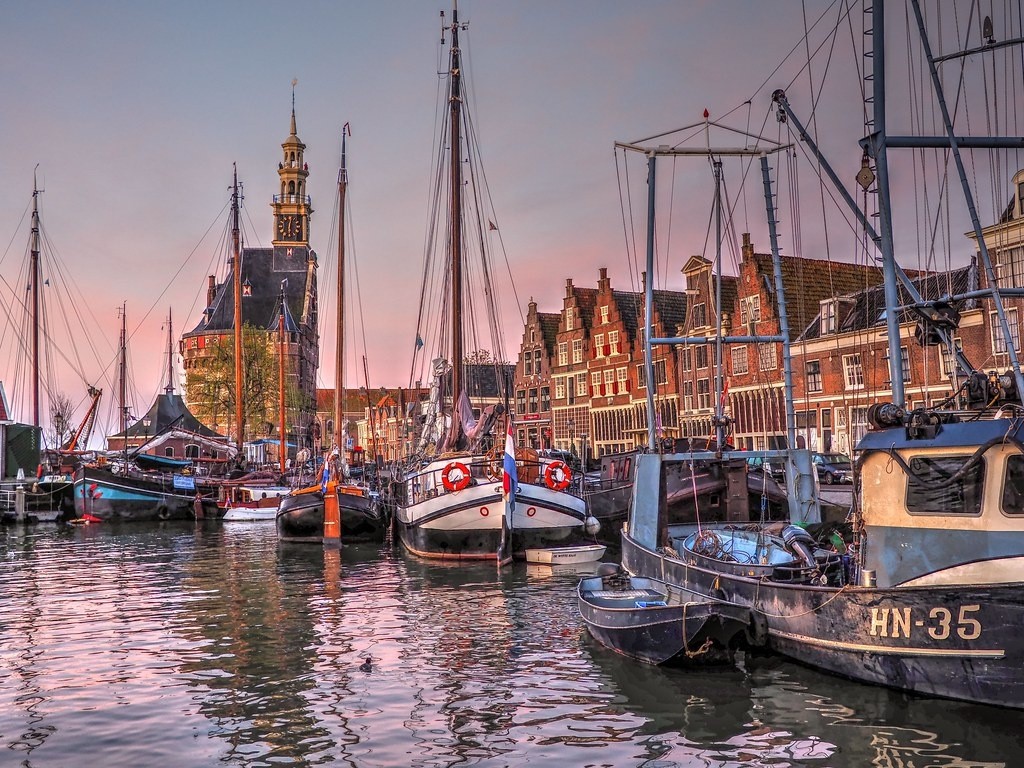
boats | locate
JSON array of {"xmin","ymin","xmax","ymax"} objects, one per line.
[
  {"xmin": 568, "ymin": 435, "xmax": 790, "ymax": 549},
  {"xmin": 577, "ymin": 572, "xmax": 751, "ymax": 665},
  {"xmin": 526, "ymin": 546, "xmax": 608, "ymax": 563},
  {"xmin": 526, "ymin": 561, "xmax": 604, "ymax": 578},
  {"xmin": 619, "ymin": 0, "xmax": 1024, "ymax": 708}
]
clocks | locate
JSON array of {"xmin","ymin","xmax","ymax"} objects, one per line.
[{"xmin": 277, "ymin": 215, "xmax": 303, "ymax": 241}]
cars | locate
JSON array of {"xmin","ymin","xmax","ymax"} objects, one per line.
[
  {"xmin": 746, "ymin": 457, "xmax": 786, "ymax": 483},
  {"xmin": 813, "ymin": 452, "xmax": 856, "ymax": 485}
]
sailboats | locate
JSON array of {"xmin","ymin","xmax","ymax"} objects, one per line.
[{"xmin": 0, "ymin": 0, "xmax": 588, "ymax": 566}]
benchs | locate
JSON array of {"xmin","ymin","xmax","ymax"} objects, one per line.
[{"xmin": 583, "ymin": 589, "xmax": 664, "ymax": 608}]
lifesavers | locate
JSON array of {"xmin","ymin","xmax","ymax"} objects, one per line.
[
  {"xmin": 544, "ymin": 461, "xmax": 571, "ymax": 491},
  {"xmin": 442, "ymin": 462, "xmax": 470, "ymax": 492}
]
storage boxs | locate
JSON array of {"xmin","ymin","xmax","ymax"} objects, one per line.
[{"xmin": 634, "ymin": 601, "xmax": 667, "ymax": 608}]
